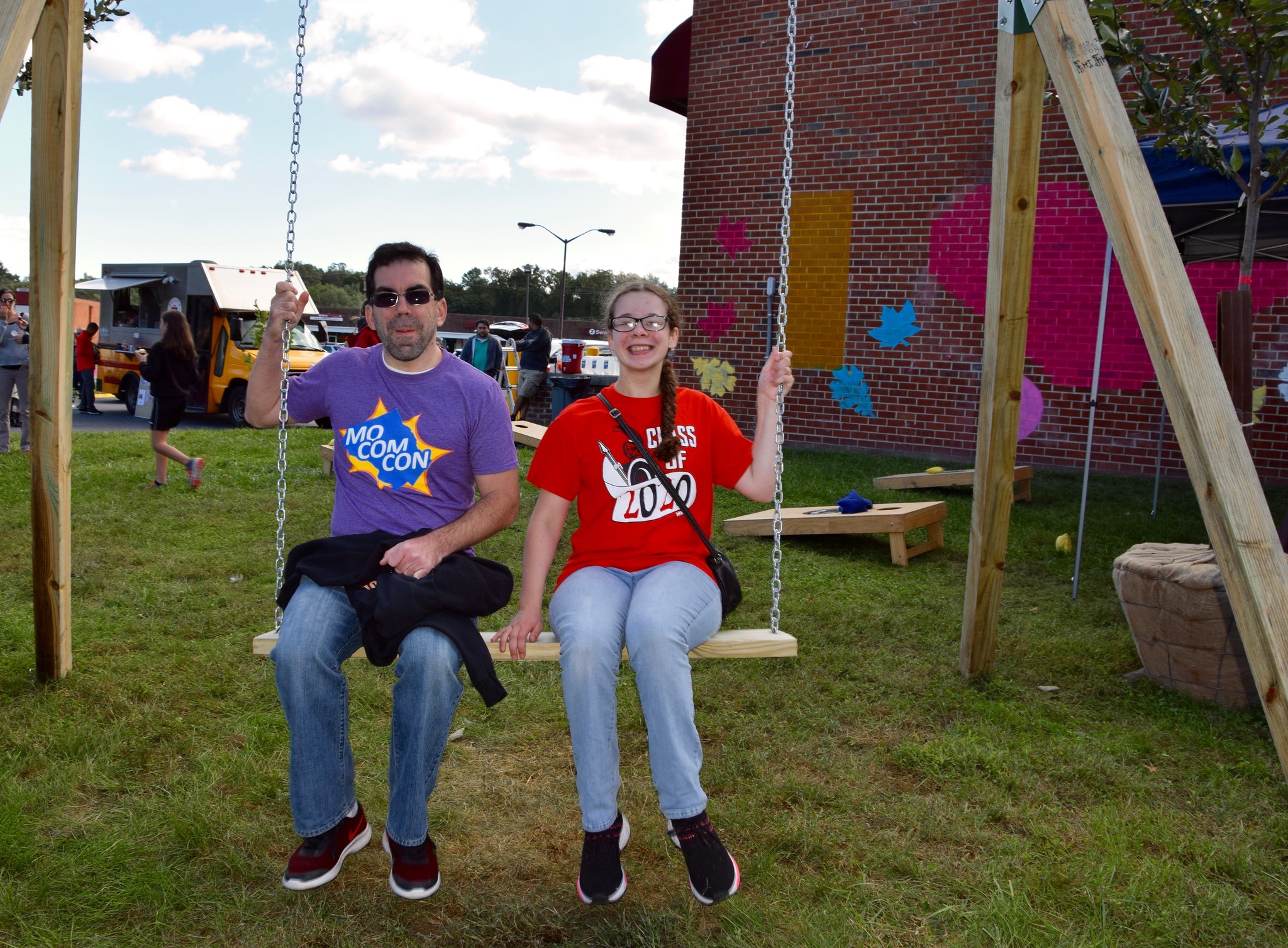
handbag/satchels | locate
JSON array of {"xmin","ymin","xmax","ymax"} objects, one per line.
[{"xmin": 705, "ymin": 547, "xmax": 743, "ymax": 619}]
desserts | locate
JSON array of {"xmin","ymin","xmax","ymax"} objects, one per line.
[
  {"xmin": 138, "ymin": 349, "xmax": 146, "ymax": 355},
  {"xmin": 18, "ymin": 316, "xmax": 22, "ymax": 320}
]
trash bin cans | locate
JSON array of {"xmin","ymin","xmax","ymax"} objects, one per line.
[{"xmin": 549, "ymin": 375, "xmax": 592, "ymax": 422}]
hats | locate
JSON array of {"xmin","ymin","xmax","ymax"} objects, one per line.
[{"xmin": 74, "ymin": 327, "xmax": 85, "ymax": 333}]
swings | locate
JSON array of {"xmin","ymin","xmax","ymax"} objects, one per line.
[{"xmin": 253, "ymin": 1, "xmax": 799, "ymax": 661}]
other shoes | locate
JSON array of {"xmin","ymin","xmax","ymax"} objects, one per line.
[
  {"xmin": 23, "ymin": 450, "xmax": 30, "ymax": 454},
  {"xmin": 78, "ymin": 407, "xmax": 104, "ymax": 415}
]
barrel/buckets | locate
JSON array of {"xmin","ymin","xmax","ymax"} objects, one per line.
[
  {"xmin": 580, "ymin": 353, "xmax": 620, "ymax": 375},
  {"xmin": 559, "ymin": 339, "xmax": 586, "ymax": 374}
]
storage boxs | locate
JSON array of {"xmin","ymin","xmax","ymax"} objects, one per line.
[
  {"xmin": 118, "ymin": 312, "xmax": 130, "ymax": 324},
  {"xmin": 592, "ymin": 356, "xmax": 604, "ymax": 375},
  {"xmin": 580, "ymin": 355, "xmax": 593, "ymax": 374},
  {"xmin": 603, "ymin": 356, "xmax": 620, "ymax": 376},
  {"xmin": 127, "ymin": 313, "xmax": 139, "ymax": 325}
]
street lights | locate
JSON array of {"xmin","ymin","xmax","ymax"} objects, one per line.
[
  {"xmin": 524, "ymin": 264, "xmax": 532, "ymax": 325},
  {"xmin": 518, "ymin": 221, "xmax": 615, "ymax": 372}
]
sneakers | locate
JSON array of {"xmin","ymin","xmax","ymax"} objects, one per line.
[
  {"xmin": 145, "ymin": 481, "xmax": 169, "ymax": 490},
  {"xmin": 184, "ymin": 457, "xmax": 206, "ymax": 491},
  {"xmin": 281, "ymin": 796, "xmax": 371, "ymax": 891},
  {"xmin": 666, "ymin": 809, "xmax": 741, "ymax": 906},
  {"xmin": 382, "ymin": 824, "xmax": 442, "ymax": 901},
  {"xmin": 576, "ymin": 809, "xmax": 631, "ymax": 905}
]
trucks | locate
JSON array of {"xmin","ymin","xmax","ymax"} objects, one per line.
[{"xmin": 74, "ymin": 259, "xmax": 332, "ymax": 430}]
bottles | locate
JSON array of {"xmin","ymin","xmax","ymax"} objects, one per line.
[{"xmin": 122, "ymin": 340, "xmax": 133, "ymax": 352}]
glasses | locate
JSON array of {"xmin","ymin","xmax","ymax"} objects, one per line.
[
  {"xmin": 368, "ymin": 290, "xmax": 438, "ymax": 308},
  {"xmin": 0, "ymin": 298, "xmax": 14, "ymax": 304},
  {"xmin": 608, "ymin": 315, "xmax": 670, "ymax": 332}
]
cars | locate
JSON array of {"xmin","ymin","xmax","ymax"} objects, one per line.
[{"xmin": 548, "ymin": 338, "xmax": 612, "ymax": 372}]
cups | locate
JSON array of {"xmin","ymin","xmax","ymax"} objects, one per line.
[{"xmin": 549, "ymin": 365, "xmax": 555, "ymax": 373}]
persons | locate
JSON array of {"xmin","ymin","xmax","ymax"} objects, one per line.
[
  {"xmin": 491, "ymin": 284, "xmax": 795, "ymax": 905},
  {"xmin": 0, "ymin": 288, "xmax": 206, "ymax": 492},
  {"xmin": 244, "ymin": 242, "xmax": 520, "ymax": 898},
  {"xmin": 346, "ymin": 311, "xmax": 551, "ymax": 421}
]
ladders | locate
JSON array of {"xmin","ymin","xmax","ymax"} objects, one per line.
[{"xmin": 497, "ymin": 338, "xmax": 521, "ymax": 421}]
